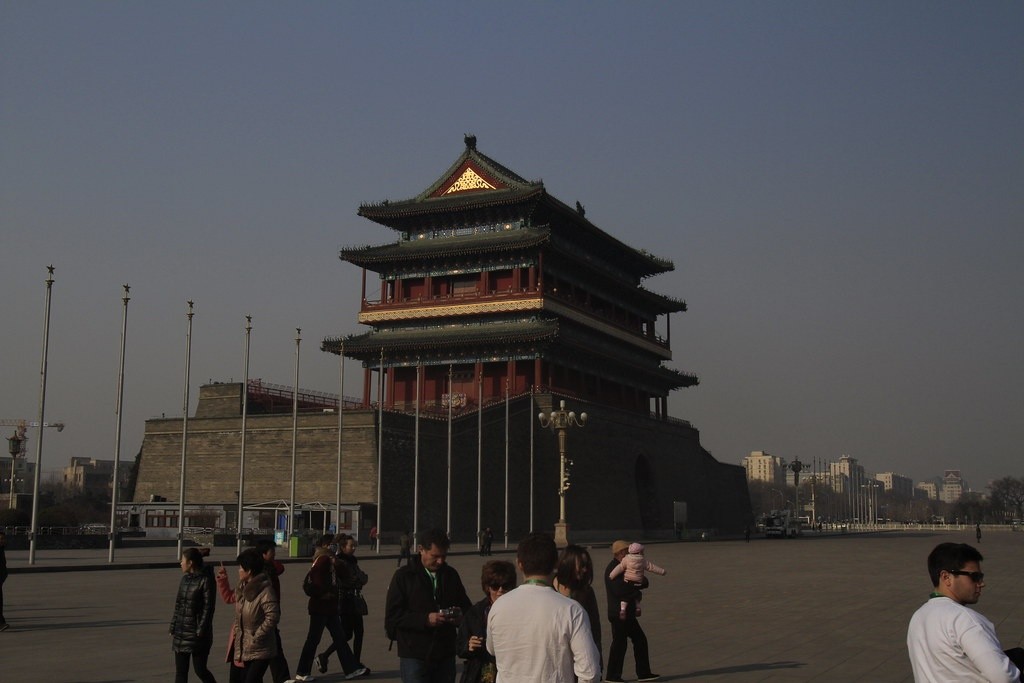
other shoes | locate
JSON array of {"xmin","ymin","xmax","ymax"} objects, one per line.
[
  {"xmin": 0, "ymin": 623, "xmax": 9, "ymax": 631},
  {"xmin": 296, "ymin": 673, "xmax": 317, "ymax": 682},
  {"xmin": 345, "ymin": 668, "xmax": 371, "ymax": 681},
  {"xmin": 638, "ymin": 674, "xmax": 660, "ymax": 681},
  {"xmin": 605, "ymin": 678, "xmax": 625, "ymax": 683},
  {"xmin": 620, "ymin": 610, "xmax": 626, "ymax": 620},
  {"xmin": 359, "ymin": 664, "xmax": 370, "ymax": 677},
  {"xmin": 315, "ymin": 655, "xmax": 327, "ymax": 673},
  {"xmin": 284, "ymin": 679, "xmax": 295, "ymax": 683},
  {"xmin": 634, "ymin": 607, "xmax": 641, "ymax": 617}
]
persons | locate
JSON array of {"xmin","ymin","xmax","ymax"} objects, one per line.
[
  {"xmin": 370, "ymin": 526, "xmax": 376, "ymax": 550},
  {"xmin": 605, "ymin": 541, "xmax": 660, "ymax": 683},
  {"xmin": 385, "ymin": 527, "xmax": 472, "ymax": 683},
  {"xmin": 257, "ymin": 539, "xmax": 298, "ymax": 683},
  {"xmin": 486, "ymin": 532, "xmax": 601, "ymax": 683},
  {"xmin": 551, "ymin": 545, "xmax": 603, "ymax": 683},
  {"xmin": 609, "ymin": 543, "xmax": 667, "ymax": 620},
  {"xmin": 480, "ymin": 528, "xmax": 493, "ymax": 556},
  {"xmin": 976, "ymin": 524, "xmax": 981, "ymax": 542},
  {"xmin": 168, "ymin": 548, "xmax": 216, "ymax": 683},
  {"xmin": 315, "ymin": 532, "xmax": 371, "ymax": 673},
  {"xmin": 745, "ymin": 525, "xmax": 750, "ymax": 543},
  {"xmin": 215, "ymin": 548, "xmax": 282, "ymax": 683},
  {"xmin": 455, "ymin": 560, "xmax": 517, "ymax": 683},
  {"xmin": 0, "ymin": 534, "xmax": 9, "ymax": 632},
  {"xmin": 397, "ymin": 530, "xmax": 412, "ymax": 567},
  {"xmin": 907, "ymin": 542, "xmax": 1021, "ymax": 683},
  {"xmin": 296, "ymin": 533, "xmax": 366, "ymax": 681}
]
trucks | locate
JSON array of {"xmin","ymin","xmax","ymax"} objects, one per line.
[{"xmin": 765, "ymin": 510, "xmax": 798, "ymax": 538}]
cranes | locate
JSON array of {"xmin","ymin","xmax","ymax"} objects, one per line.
[{"xmin": 0, "ymin": 419, "xmax": 65, "ymax": 492}]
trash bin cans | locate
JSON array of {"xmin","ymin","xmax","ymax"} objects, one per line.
[
  {"xmin": 307, "ymin": 536, "xmax": 313, "ymax": 557},
  {"xmin": 291, "ymin": 535, "xmax": 307, "ymax": 558}
]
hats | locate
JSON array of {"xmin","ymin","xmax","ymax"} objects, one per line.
[
  {"xmin": 613, "ymin": 540, "xmax": 630, "ymax": 554},
  {"xmin": 629, "ymin": 543, "xmax": 644, "ymax": 553}
]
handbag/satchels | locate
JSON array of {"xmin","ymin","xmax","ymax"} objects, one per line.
[{"xmin": 348, "ymin": 596, "xmax": 368, "ymax": 620}]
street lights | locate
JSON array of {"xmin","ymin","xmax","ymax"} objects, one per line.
[
  {"xmin": 4, "ymin": 429, "xmax": 26, "ymax": 529},
  {"xmin": 538, "ymin": 400, "xmax": 588, "ymax": 548}
]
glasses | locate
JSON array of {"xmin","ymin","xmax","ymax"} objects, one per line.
[
  {"xmin": 949, "ymin": 569, "xmax": 984, "ymax": 582},
  {"xmin": 490, "ymin": 582, "xmax": 511, "ymax": 591}
]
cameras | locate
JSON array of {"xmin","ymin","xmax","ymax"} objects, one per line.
[{"xmin": 440, "ymin": 608, "xmax": 460, "ymax": 618}]
